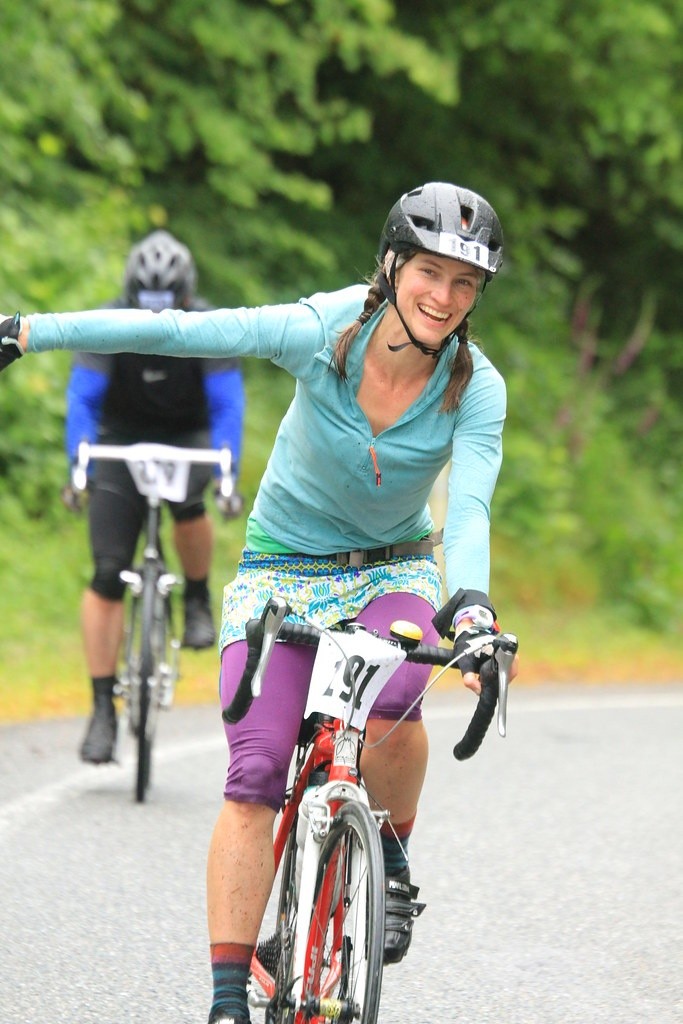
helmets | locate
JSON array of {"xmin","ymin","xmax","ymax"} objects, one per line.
[
  {"xmin": 122, "ymin": 228, "xmax": 199, "ymax": 310},
  {"xmin": 378, "ymin": 181, "xmax": 507, "ymax": 275}
]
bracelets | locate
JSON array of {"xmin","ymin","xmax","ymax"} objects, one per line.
[{"xmin": 431, "ymin": 587, "xmax": 498, "ymax": 641}]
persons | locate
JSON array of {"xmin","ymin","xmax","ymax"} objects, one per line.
[
  {"xmin": 61, "ymin": 229, "xmax": 244, "ymax": 763},
  {"xmin": 0, "ymin": 183, "xmax": 517, "ymax": 1024}
]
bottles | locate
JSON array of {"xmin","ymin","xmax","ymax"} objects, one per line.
[{"xmin": 293, "ymin": 771, "xmax": 329, "ymax": 901}]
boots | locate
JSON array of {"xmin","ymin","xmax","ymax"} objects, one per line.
[
  {"xmin": 80, "ymin": 675, "xmax": 121, "ymax": 764},
  {"xmin": 182, "ymin": 574, "xmax": 216, "ymax": 650}
]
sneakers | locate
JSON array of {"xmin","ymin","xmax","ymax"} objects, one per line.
[{"xmin": 364, "ymin": 861, "xmax": 427, "ymax": 965}]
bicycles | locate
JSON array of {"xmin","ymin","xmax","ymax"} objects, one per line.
[
  {"xmin": 222, "ymin": 597, "xmax": 519, "ymax": 1024},
  {"xmin": 72, "ymin": 439, "xmax": 233, "ymax": 800}
]
